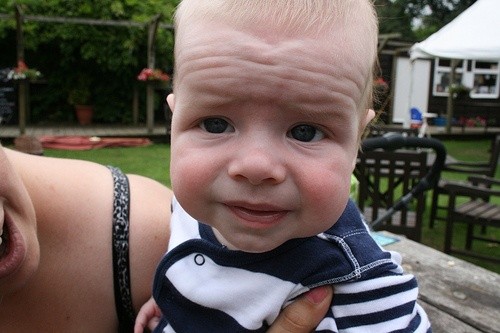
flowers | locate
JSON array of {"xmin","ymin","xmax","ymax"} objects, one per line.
[
  {"xmin": 7, "ymin": 60, "xmax": 40, "ymax": 79},
  {"xmin": 374, "ymin": 78, "xmax": 388, "ymax": 91},
  {"xmin": 137, "ymin": 67, "xmax": 170, "ymax": 81}
]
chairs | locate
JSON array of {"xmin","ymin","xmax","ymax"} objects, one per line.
[{"xmin": 352, "ymin": 123, "xmax": 500, "ymax": 268}]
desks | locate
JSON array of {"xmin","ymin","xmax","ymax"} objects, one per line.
[{"xmin": 373, "ymin": 230, "xmax": 500, "ymax": 333}]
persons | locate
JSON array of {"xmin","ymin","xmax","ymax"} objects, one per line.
[
  {"xmin": 0, "ymin": 143, "xmax": 332, "ymax": 333},
  {"xmin": 134, "ymin": 0, "xmax": 433, "ymax": 333}
]
